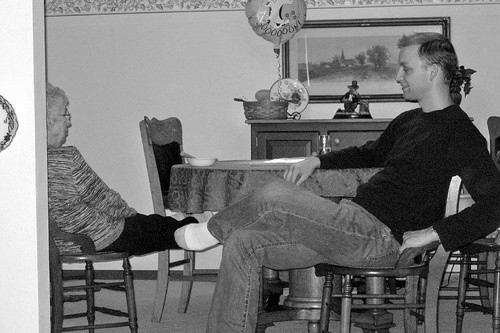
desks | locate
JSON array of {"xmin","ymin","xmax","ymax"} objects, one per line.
[{"xmin": 166, "ymin": 160, "xmax": 392, "ymax": 333}]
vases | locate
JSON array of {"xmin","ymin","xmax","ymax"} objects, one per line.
[{"xmin": 451, "ymin": 85, "xmax": 462, "ymax": 106}]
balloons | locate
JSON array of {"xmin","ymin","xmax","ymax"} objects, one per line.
[{"xmin": 245, "ymin": 0, "xmax": 307, "ymax": 52}]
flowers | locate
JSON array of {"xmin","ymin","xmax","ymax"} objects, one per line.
[{"xmin": 450, "ymin": 66, "xmax": 477, "ymax": 97}]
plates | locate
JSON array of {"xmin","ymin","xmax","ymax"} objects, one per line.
[{"xmin": 269, "ymin": 78, "xmax": 309, "ymax": 118}]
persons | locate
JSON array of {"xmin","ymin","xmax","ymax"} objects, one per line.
[
  {"xmin": 46, "ymin": 83, "xmax": 219, "ymax": 256},
  {"xmin": 174, "ymin": 32, "xmax": 500, "ymax": 333}
]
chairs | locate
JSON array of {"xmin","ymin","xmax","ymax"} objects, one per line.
[
  {"xmin": 48, "ymin": 216, "xmax": 139, "ymax": 333},
  {"xmin": 139, "ymin": 116, "xmax": 219, "ymax": 324},
  {"xmin": 315, "ymin": 116, "xmax": 500, "ymax": 333}
]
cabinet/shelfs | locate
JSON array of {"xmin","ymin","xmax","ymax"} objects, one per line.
[{"xmin": 246, "ymin": 118, "xmax": 393, "ymax": 160}]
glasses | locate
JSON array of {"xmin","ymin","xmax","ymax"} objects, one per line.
[{"xmin": 52, "ymin": 110, "xmax": 72, "ymax": 121}]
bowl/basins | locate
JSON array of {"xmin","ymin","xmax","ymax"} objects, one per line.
[{"xmin": 185, "ymin": 157, "xmax": 217, "ymax": 167}]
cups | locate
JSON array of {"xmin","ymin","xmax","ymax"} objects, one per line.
[{"xmin": 317, "ymin": 135, "xmax": 332, "ymax": 156}]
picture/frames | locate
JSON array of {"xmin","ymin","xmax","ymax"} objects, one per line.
[
  {"xmin": 0, "ymin": 95, "xmax": 19, "ymax": 152},
  {"xmin": 282, "ymin": 17, "xmax": 452, "ymax": 103}
]
